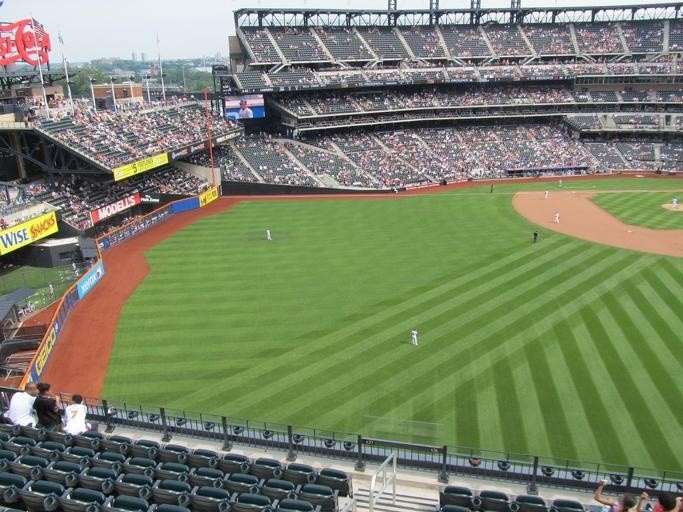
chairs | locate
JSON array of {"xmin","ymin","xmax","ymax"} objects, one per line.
[{"xmin": 0, "ymin": 424, "xmax": 586, "ymax": 511}]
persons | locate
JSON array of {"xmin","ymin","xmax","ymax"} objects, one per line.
[
  {"xmin": 243, "ymin": 17, "xmax": 683, "ymax": 195},
  {"xmin": 592, "ymin": 480, "xmax": 637, "ymax": 512},
  {"xmin": 3, "ymin": 382, "xmax": 39, "ymax": 428},
  {"xmin": 31, "ymin": 382, "xmax": 63, "ymax": 432},
  {"xmin": 650, "ymin": 492, "xmax": 682, "ymax": 512},
  {"xmin": 13, "ymin": 253, "xmax": 99, "ymax": 319},
  {"xmin": 553, "ymin": 212, "xmax": 559, "ymax": 223},
  {"xmin": 63, "ymin": 393, "xmax": 98, "ymax": 435},
  {"xmin": 532, "ymin": 230, "xmax": 538, "ymax": 244},
  {"xmin": 0, "ymin": 76, "xmax": 243, "ymax": 240},
  {"xmin": 265, "ymin": 229, "xmax": 272, "ymax": 240},
  {"xmin": 626, "ymin": 492, "xmax": 648, "ymax": 512},
  {"xmin": 543, "ymin": 188, "xmax": 549, "ymax": 200},
  {"xmin": 669, "ymin": 196, "xmax": 677, "ymax": 209},
  {"xmin": 410, "ymin": 328, "xmax": 418, "ymax": 346}
]
